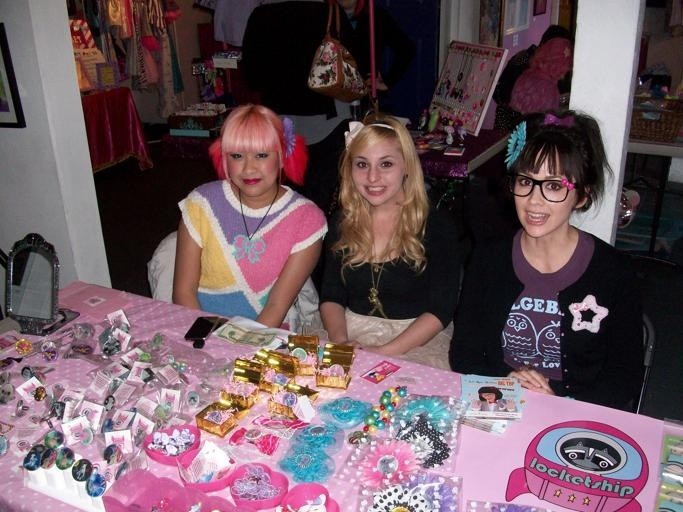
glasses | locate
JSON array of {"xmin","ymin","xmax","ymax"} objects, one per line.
[{"xmin": 506, "ymin": 173, "xmax": 577, "ymax": 202}]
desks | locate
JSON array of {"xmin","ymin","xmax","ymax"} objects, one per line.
[
  {"xmin": 618, "ymin": 135, "xmax": 683, "ymax": 270},
  {"xmin": 78, "ymin": 85, "xmax": 157, "ymax": 178},
  {"xmin": 411, "ymin": 128, "xmax": 513, "ymax": 246},
  {"xmin": 1, "ymin": 280, "xmax": 683, "ymax": 512}
]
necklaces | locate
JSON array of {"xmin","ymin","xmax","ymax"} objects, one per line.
[
  {"xmin": 232, "ymin": 181, "xmax": 279, "ymax": 263},
  {"xmin": 367, "ymin": 241, "xmax": 394, "ymax": 318},
  {"xmin": 449, "ymin": 48, "xmax": 476, "ymax": 103}
]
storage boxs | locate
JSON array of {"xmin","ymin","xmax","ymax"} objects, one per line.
[
  {"xmin": 159, "ymin": 134, "xmax": 219, "ymax": 159},
  {"xmin": 169, "ymin": 107, "xmax": 233, "ymax": 137}
]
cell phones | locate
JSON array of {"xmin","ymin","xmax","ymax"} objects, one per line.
[{"xmin": 185, "ymin": 316, "xmax": 221, "ymax": 342}]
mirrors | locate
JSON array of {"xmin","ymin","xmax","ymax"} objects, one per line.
[{"xmin": 4, "ymin": 233, "xmax": 81, "ymax": 336}]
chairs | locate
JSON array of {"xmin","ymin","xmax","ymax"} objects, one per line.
[{"xmin": 632, "ymin": 313, "xmax": 656, "ymax": 413}]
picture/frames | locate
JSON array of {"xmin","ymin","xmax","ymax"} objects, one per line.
[
  {"xmin": 533, "ymin": 0, "xmax": 548, "ymax": 16},
  {"xmin": 503, "ymin": 0, "xmax": 530, "ymax": 37},
  {"xmin": 0, "ymin": 22, "xmax": 27, "ymax": 128}
]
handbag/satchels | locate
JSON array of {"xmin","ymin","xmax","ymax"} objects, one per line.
[{"xmin": 307, "ymin": 37, "xmax": 367, "ymax": 103}]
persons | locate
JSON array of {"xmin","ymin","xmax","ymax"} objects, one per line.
[
  {"xmin": 469, "ymin": 385, "xmax": 518, "ymax": 412},
  {"xmin": 299, "ymin": 115, "xmax": 467, "ymax": 372},
  {"xmin": 170, "ymin": 104, "xmax": 330, "ymax": 333},
  {"xmin": 447, "ymin": 107, "xmax": 645, "ymax": 411},
  {"xmin": 509, "ymin": 37, "xmax": 574, "ymax": 114},
  {"xmin": 491, "ymin": 24, "xmax": 569, "ymax": 133},
  {"xmin": 239, "ymin": 0, "xmax": 369, "ymax": 211}
]
